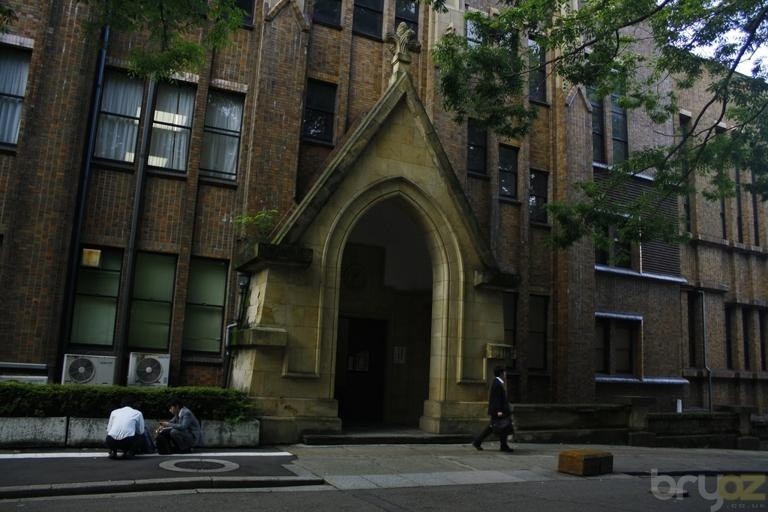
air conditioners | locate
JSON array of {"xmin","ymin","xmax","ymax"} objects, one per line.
[
  {"xmin": 125, "ymin": 351, "xmax": 172, "ymax": 388},
  {"xmin": 61, "ymin": 352, "xmax": 120, "ymax": 385}
]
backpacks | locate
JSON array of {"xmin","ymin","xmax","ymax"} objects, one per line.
[{"xmin": 155, "ymin": 430, "xmax": 177, "ymax": 454}]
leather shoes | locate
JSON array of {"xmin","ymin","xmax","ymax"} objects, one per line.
[
  {"xmin": 500, "ymin": 445, "xmax": 513, "ymax": 452},
  {"xmin": 109, "ymin": 450, "xmax": 117, "ymax": 459},
  {"xmin": 123, "ymin": 450, "xmax": 136, "ymax": 459},
  {"xmin": 472, "ymin": 440, "xmax": 483, "ymax": 451}
]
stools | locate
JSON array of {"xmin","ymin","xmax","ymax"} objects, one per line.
[{"xmin": 558, "ymin": 448, "xmax": 614, "ymax": 477}]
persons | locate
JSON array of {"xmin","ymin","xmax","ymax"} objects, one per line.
[
  {"xmin": 472, "ymin": 363, "xmax": 517, "ymax": 453},
  {"xmin": 153, "ymin": 397, "xmax": 201, "ymax": 453},
  {"xmin": 101, "ymin": 395, "xmax": 145, "ymax": 460}
]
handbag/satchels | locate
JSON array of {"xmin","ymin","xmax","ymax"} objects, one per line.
[{"xmin": 488, "ymin": 411, "xmax": 516, "ymax": 437}]
